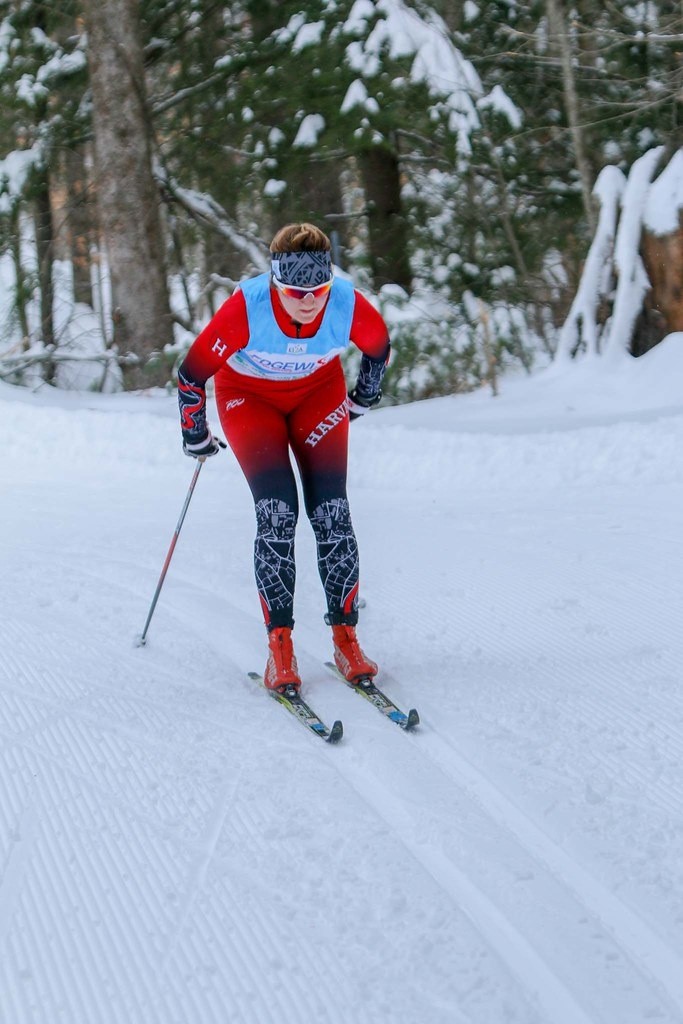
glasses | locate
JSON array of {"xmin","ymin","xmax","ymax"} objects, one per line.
[{"xmin": 274, "ymin": 281, "xmax": 332, "ymax": 299}]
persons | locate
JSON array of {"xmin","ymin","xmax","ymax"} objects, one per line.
[{"xmin": 177, "ymin": 224, "xmax": 392, "ymax": 693}]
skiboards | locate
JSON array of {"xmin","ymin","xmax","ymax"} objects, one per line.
[{"xmin": 247, "ymin": 660, "xmax": 421, "ymax": 743}]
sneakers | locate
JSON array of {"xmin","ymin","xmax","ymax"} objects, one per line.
[
  {"xmin": 264, "ymin": 627, "xmax": 302, "ymax": 692},
  {"xmin": 331, "ymin": 623, "xmax": 378, "ymax": 681}
]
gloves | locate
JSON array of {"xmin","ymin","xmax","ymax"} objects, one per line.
[
  {"xmin": 347, "ymin": 389, "xmax": 382, "ymax": 421},
  {"xmin": 183, "ymin": 431, "xmax": 219, "ymax": 459}
]
